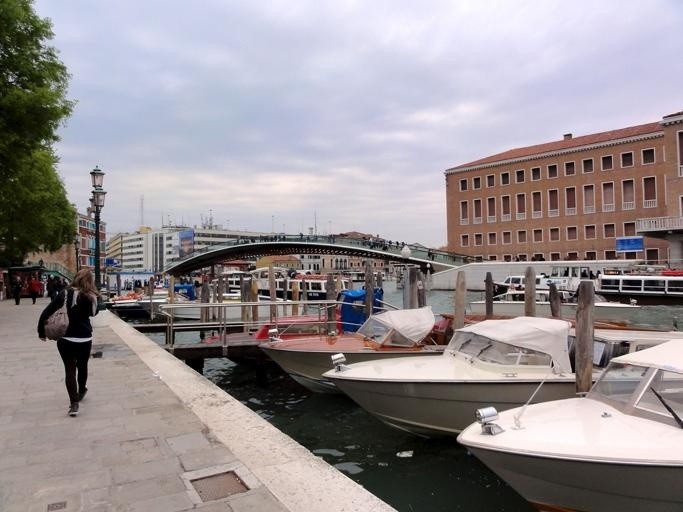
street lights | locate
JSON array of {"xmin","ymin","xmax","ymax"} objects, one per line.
[
  {"xmin": 89, "ymin": 166, "xmax": 107, "ymax": 300},
  {"xmin": 426, "ymin": 263, "xmax": 431, "ymax": 290},
  {"xmin": 74, "ymin": 239, "xmax": 80, "ymax": 271}
]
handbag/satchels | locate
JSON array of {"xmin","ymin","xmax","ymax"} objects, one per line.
[{"xmin": 44, "ymin": 290, "xmax": 70, "ymax": 341}]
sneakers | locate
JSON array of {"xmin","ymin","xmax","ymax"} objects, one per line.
[{"xmin": 66, "ymin": 385, "xmax": 90, "ymax": 417}]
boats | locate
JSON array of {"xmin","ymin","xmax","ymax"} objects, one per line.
[
  {"xmin": 469, "ymin": 266, "xmax": 642, "ymax": 320},
  {"xmin": 111, "ymin": 287, "xmax": 304, "ymax": 319}
]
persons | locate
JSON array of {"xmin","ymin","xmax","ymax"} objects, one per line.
[
  {"xmin": 38, "ymin": 269, "xmax": 100, "ymax": 415},
  {"xmin": 493, "ymin": 285, "xmax": 525, "ymax": 301},
  {"xmin": 12, "ymin": 275, "xmax": 68, "ymax": 304},
  {"xmin": 427, "ymin": 249, "xmax": 433, "ymax": 260},
  {"xmin": 193, "ymin": 280, "xmax": 200, "ymax": 297},
  {"xmin": 363, "ymin": 237, "xmax": 405, "ymax": 251},
  {"xmin": 564, "ymin": 267, "xmax": 601, "ymax": 279},
  {"xmin": 240, "ymin": 235, "xmax": 286, "ymax": 244},
  {"xmin": 300, "ymin": 233, "xmax": 336, "ymax": 243}
]
